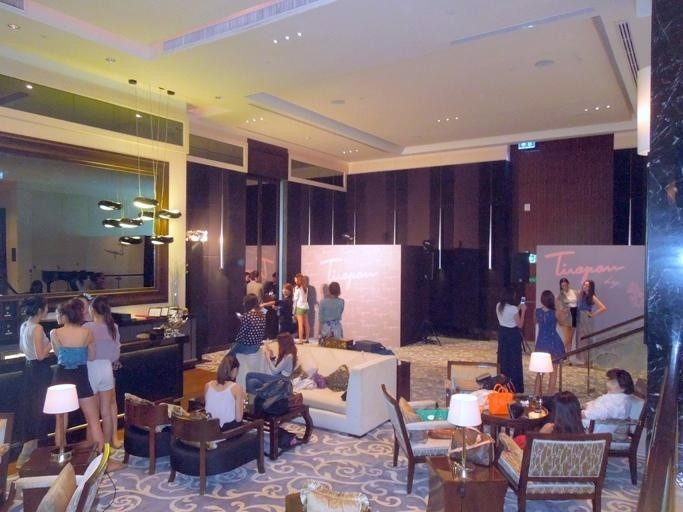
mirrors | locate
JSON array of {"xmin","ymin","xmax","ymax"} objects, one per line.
[{"xmin": 1, "ymin": 130, "xmax": 168, "ymax": 312}]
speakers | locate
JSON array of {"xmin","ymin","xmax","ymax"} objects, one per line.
[
  {"xmin": 510, "ymin": 253, "xmax": 531, "ymax": 285},
  {"xmin": 415, "ymin": 250, "xmax": 437, "ymax": 281}
]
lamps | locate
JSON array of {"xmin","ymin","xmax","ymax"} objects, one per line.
[
  {"xmin": 635, "ymin": 62, "xmax": 653, "ymax": 158},
  {"xmin": 185, "ymin": 228, "xmax": 209, "ymax": 245},
  {"xmin": 97, "ymin": 167, "xmax": 154, "ymax": 245},
  {"xmin": 119, "ymin": 79, "xmax": 182, "ymax": 246}
]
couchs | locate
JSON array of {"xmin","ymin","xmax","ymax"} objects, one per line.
[{"xmin": 233, "ymin": 339, "xmax": 399, "ymax": 439}]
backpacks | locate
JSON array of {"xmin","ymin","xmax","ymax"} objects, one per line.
[{"xmin": 254, "ymin": 378, "xmax": 293, "ymax": 416}]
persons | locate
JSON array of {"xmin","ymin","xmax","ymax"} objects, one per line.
[
  {"xmin": 50, "ymin": 299, "xmax": 105, "ymax": 457},
  {"xmin": 230, "ymin": 271, "xmax": 346, "ymax": 395},
  {"xmin": 533, "ymin": 291, "xmax": 566, "ymax": 396},
  {"xmin": 15, "ymin": 294, "xmax": 56, "ymax": 471},
  {"xmin": 580, "ymin": 369, "xmax": 635, "ymax": 441},
  {"xmin": 495, "ymin": 286, "xmax": 527, "ymax": 393},
  {"xmin": 512, "ymin": 391, "xmax": 588, "ymax": 452},
  {"xmin": 81, "ymin": 296, "xmax": 123, "ymax": 453},
  {"xmin": 203, "ymin": 354, "xmax": 246, "ymax": 440},
  {"xmin": 576, "ymin": 281, "xmax": 608, "ymax": 365},
  {"xmin": 90, "ymin": 272, "xmax": 109, "ymax": 291},
  {"xmin": 28, "ymin": 280, "xmax": 46, "ymax": 295},
  {"xmin": 558, "ymin": 279, "xmax": 578, "ymax": 357},
  {"xmin": 74, "ymin": 293, "xmax": 91, "ymax": 324}
]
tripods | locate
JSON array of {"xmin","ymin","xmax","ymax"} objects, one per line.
[
  {"xmin": 506, "ymin": 284, "xmax": 533, "ymax": 356},
  {"xmin": 408, "ymin": 281, "xmax": 442, "ymax": 347}
]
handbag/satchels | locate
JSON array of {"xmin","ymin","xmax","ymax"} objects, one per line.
[
  {"xmin": 327, "ymin": 365, "xmax": 350, "ymax": 391},
  {"xmin": 289, "ymin": 393, "xmax": 303, "ymax": 412},
  {"xmin": 489, "ymin": 384, "xmax": 514, "ymax": 415},
  {"xmin": 450, "ymin": 427, "xmax": 494, "ymax": 466}
]
